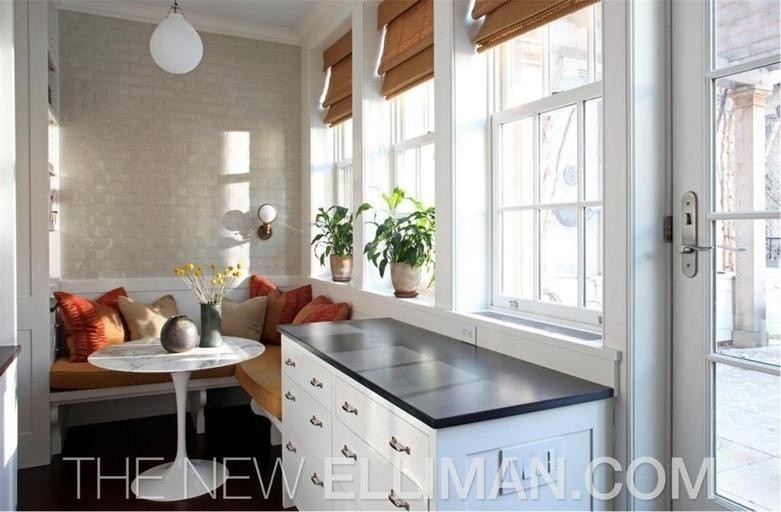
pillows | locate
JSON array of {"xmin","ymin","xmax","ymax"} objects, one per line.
[
  {"xmin": 210, "ymin": 294, "xmax": 268, "ymax": 342},
  {"xmin": 249, "ymin": 274, "xmax": 312, "ymax": 345},
  {"xmin": 53, "ymin": 287, "xmax": 130, "ymax": 362},
  {"xmin": 291, "ymin": 295, "xmax": 349, "ymax": 323},
  {"xmin": 118, "ymin": 294, "xmax": 179, "ymax": 341}
]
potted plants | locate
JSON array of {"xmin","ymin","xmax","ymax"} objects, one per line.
[
  {"xmin": 356, "ymin": 185, "xmax": 436, "ymax": 298},
  {"xmin": 309, "ymin": 205, "xmax": 353, "ymax": 282}
]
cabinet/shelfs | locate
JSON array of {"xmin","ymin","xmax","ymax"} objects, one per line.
[
  {"xmin": 275, "ymin": 317, "xmax": 615, "ymax": 511},
  {"xmin": 15, "ymin": 0, "xmax": 60, "ymax": 468}
]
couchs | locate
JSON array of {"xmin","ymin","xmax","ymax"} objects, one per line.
[{"xmin": 50, "ymin": 275, "xmax": 372, "ymax": 455}]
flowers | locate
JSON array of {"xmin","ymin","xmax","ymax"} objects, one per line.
[{"xmin": 174, "ymin": 263, "xmax": 243, "ymax": 303}]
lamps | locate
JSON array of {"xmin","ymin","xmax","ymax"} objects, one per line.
[
  {"xmin": 257, "ymin": 204, "xmax": 277, "ymax": 240},
  {"xmin": 149, "ymin": 0, "xmax": 204, "ymax": 74}
]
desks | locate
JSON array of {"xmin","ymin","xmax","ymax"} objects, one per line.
[{"xmin": 0, "ymin": 346, "xmax": 22, "ymax": 376}]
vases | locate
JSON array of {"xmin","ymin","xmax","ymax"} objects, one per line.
[{"xmin": 199, "ymin": 302, "xmax": 223, "ymax": 347}]
